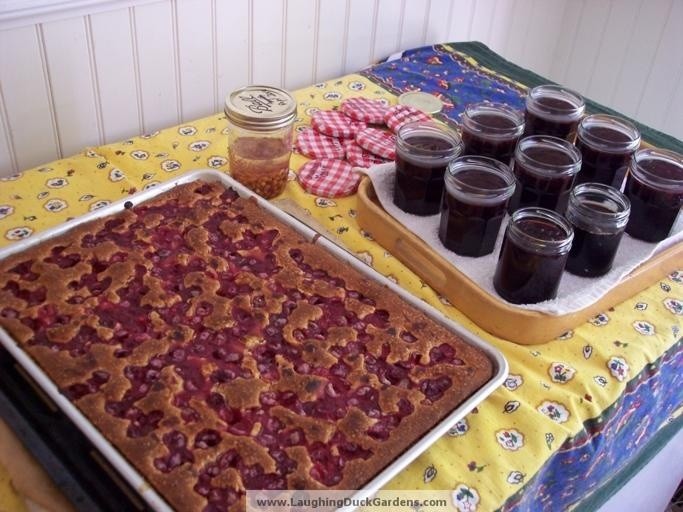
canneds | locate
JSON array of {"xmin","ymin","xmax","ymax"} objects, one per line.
[
  {"xmin": 224, "ymin": 85, "xmax": 298, "ymax": 200},
  {"xmin": 393, "ymin": 84, "xmax": 683, "ymax": 304}
]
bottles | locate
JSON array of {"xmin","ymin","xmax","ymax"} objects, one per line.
[
  {"xmin": 394, "ymin": 84, "xmax": 683, "ymax": 304},
  {"xmin": 224, "ymin": 86, "xmax": 296, "ymax": 202}
]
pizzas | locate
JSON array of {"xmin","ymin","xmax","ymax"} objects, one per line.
[{"xmin": 0, "ymin": 179, "xmax": 492, "ymax": 512}]
